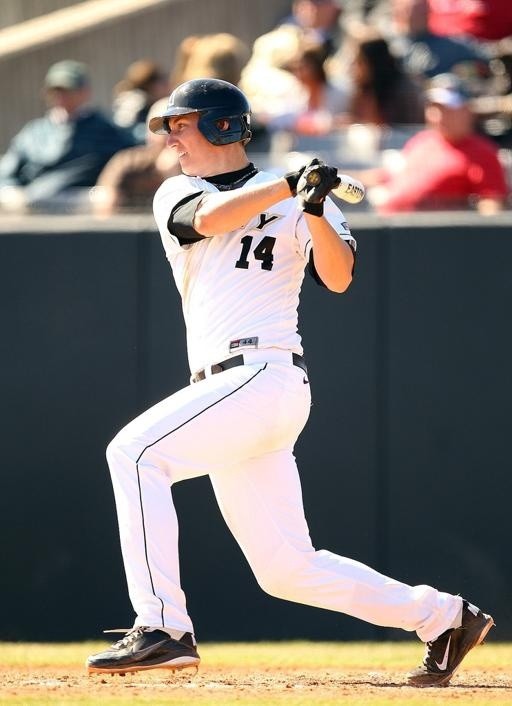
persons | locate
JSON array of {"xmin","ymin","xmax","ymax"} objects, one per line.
[
  {"xmin": 96, "ymin": 1, "xmax": 511, "ymax": 214},
  {"xmin": 88, "ymin": 77, "xmax": 495, "ymax": 688},
  {"xmin": 2, "ymin": 59, "xmax": 136, "ymax": 214}
]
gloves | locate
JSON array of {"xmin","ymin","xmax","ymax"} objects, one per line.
[
  {"xmin": 298, "ymin": 167, "xmax": 340, "ymax": 215},
  {"xmin": 283, "ymin": 161, "xmax": 342, "ymax": 197}
]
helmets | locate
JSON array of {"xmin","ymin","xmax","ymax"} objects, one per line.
[{"xmin": 149, "ymin": 78, "xmax": 251, "ymax": 149}]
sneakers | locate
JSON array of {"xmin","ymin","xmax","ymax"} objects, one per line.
[
  {"xmin": 407, "ymin": 597, "xmax": 493, "ymax": 686},
  {"xmin": 85, "ymin": 625, "xmax": 202, "ymax": 672}
]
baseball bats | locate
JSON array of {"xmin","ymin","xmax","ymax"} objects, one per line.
[{"xmin": 307, "ymin": 168, "xmax": 365, "ymax": 204}]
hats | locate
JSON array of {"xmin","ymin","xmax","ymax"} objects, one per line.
[
  {"xmin": 42, "ymin": 59, "xmax": 89, "ymax": 93},
  {"xmin": 416, "ymin": 74, "xmax": 470, "ymax": 109}
]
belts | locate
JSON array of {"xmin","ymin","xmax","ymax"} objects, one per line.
[{"xmin": 191, "ymin": 350, "xmax": 309, "ymax": 382}]
